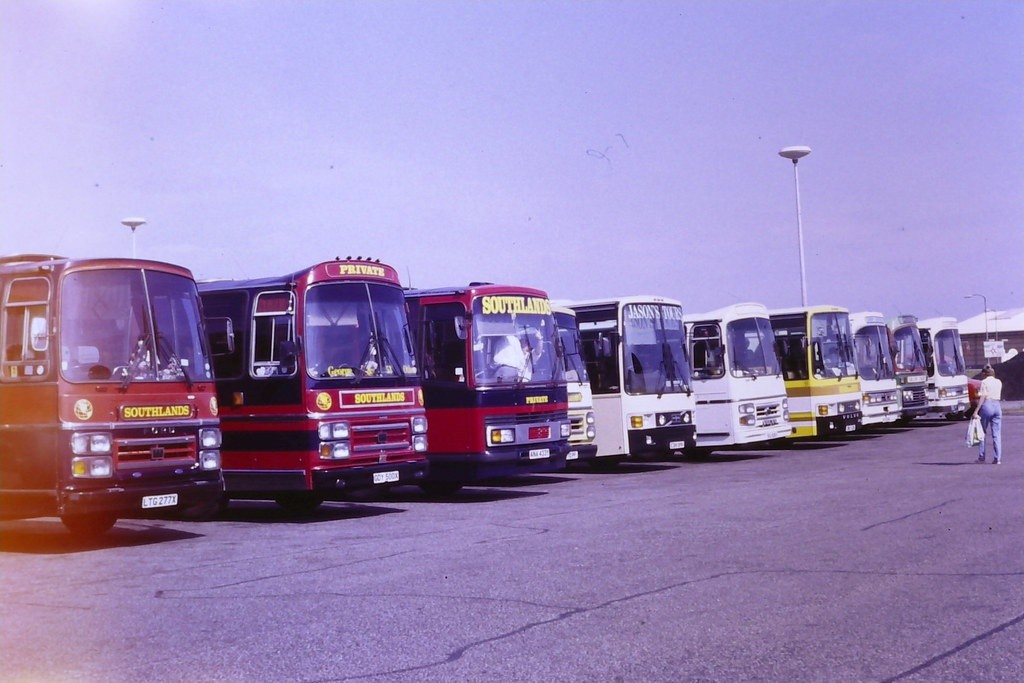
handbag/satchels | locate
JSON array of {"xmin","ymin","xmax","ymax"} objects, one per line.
[{"xmin": 965, "ymin": 415, "xmax": 986, "ymax": 449}]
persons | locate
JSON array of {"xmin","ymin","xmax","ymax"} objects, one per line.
[{"xmin": 972, "ymin": 363, "xmax": 1003, "ymax": 465}]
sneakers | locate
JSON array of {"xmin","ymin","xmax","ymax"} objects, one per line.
[
  {"xmin": 974, "ymin": 460, "xmax": 985, "ymax": 464},
  {"xmin": 992, "ymin": 461, "xmax": 1001, "ymax": 464}
]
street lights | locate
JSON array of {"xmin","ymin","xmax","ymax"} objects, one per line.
[
  {"xmin": 778, "ymin": 145, "xmax": 813, "ymax": 309},
  {"xmin": 121, "ymin": 217, "xmax": 147, "ymax": 254},
  {"xmin": 964, "ymin": 293, "xmax": 999, "ymax": 365}
]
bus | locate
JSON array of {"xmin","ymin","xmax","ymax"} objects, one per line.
[
  {"xmin": 343, "ymin": 282, "xmax": 571, "ymax": 499},
  {"xmin": 885, "ymin": 314, "xmax": 928, "ymax": 420},
  {"xmin": 1, "ymin": 255, "xmax": 225, "ymax": 538},
  {"xmin": 916, "ymin": 317, "xmax": 970, "ymax": 419},
  {"xmin": 547, "ymin": 305, "xmax": 599, "ymax": 462},
  {"xmin": 768, "ymin": 304, "xmax": 863, "ymax": 449},
  {"xmin": 683, "ymin": 303, "xmax": 791, "ymax": 459},
  {"xmin": 194, "ymin": 255, "xmax": 429, "ymax": 522},
  {"xmin": 847, "ymin": 312, "xmax": 901, "ymax": 427},
  {"xmin": 548, "ymin": 294, "xmax": 698, "ymax": 470}
]
